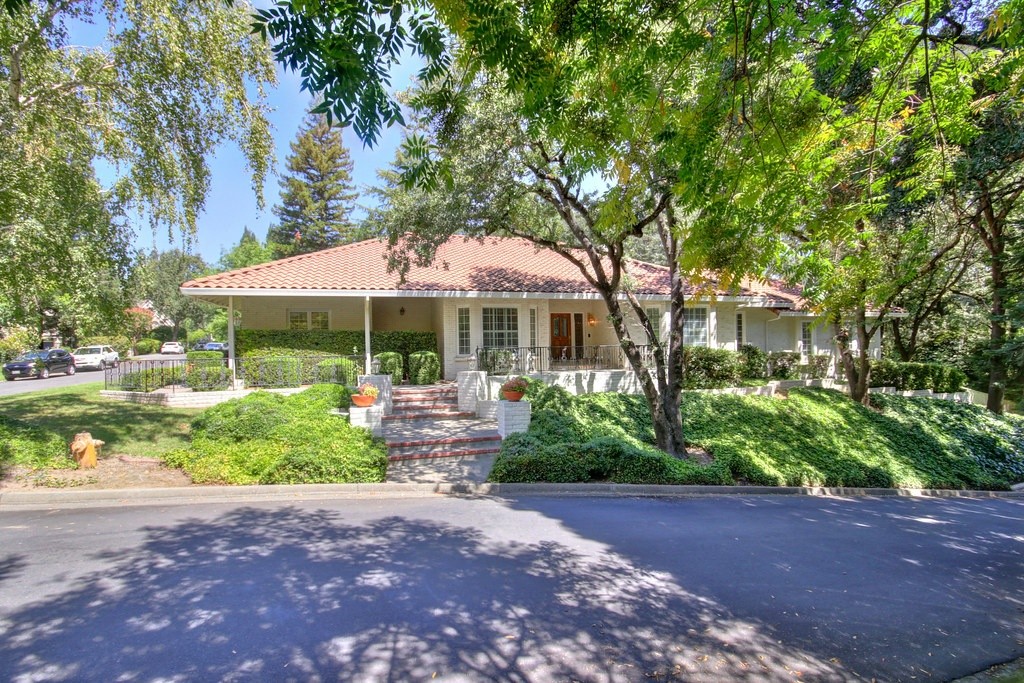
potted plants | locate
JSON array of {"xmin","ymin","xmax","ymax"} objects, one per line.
[
  {"xmin": 351, "ymin": 386, "xmax": 381, "ymax": 407},
  {"xmin": 500, "ymin": 377, "xmax": 529, "ymax": 402}
]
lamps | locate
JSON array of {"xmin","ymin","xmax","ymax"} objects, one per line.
[
  {"xmin": 400, "ymin": 306, "xmax": 405, "ymax": 315},
  {"xmin": 588, "ymin": 315, "xmax": 596, "ymax": 327}
]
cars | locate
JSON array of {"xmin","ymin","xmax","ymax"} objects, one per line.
[
  {"xmin": 204, "ymin": 342, "xmax": 230, "ymax": 351},
  {"xmin": 160, "ymin": 341, "xmax": 185, "ymax": 354}
]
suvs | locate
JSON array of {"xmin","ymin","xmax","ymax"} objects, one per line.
[
  {"xmin": 2, "ymin": 347, "xmax": 76, "ymax": 381},
  {"xmin": 69, "ymin": 343, "xmax": 122, "ymax": 372}
]
